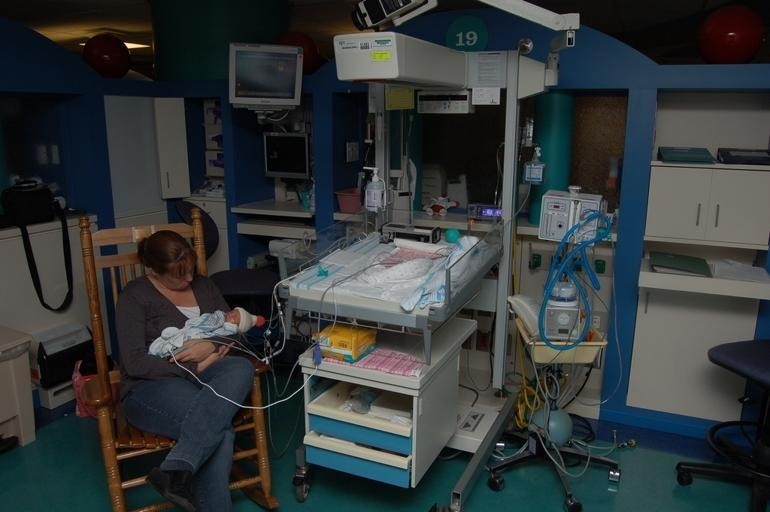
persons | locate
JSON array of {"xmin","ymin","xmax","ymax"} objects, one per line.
[
  {"xmin": 148, "ymin": 306, "xmax": 265, "ymax": 360},
  {"xmin": 116, "ymin": 231, "xmax": 256, "ymax": 512}
]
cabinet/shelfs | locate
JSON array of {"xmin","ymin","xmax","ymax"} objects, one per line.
[
  {"xmin": 231, "ymin": 198, "xmax": 316, "ymax": 240},
  {"xmin": 625, "ymin": 284, "xmax": 761, "ymax": 422},
  {"xmin": 298, "ymin": 318, "xmax": 477, "ymax": 488},
  {"xmin": 154, "ymin": 98, "xmax": 229, "ymax": 277},
  {"xmin": 643, "ymin": 161, "xmax": 770, "ymax": 251}
]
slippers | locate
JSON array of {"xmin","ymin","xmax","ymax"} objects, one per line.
[{"xmin": 149, "ymin": 468, "xmax": 199, "ymax": 512}]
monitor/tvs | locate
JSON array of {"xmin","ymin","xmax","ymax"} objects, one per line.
[
  {"xmin": 263, "ymin": 132, "xmax": 310, "ymax": 180},
  {"xmin": 229, "ymin": 43, "xmax": 304, "ymax": 111}
]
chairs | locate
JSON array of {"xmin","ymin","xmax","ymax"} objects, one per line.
[
  {"xmin": 175, "ymin": 200, "xmax": 281, "ymax": 314},
  {"xmin": 79, "ymin": 207, "xmax": 278, "ymax": 512},
  {"xmin": 676, "ymin": 340, "xmax": 770, "ymax": 512}
]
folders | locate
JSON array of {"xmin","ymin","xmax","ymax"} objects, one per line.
[
  {"xmin": 649, "ymin": 251, "xmax": 713, "ymax": 278},
  {"xmin": 658, "ymin": 146, "xmax": 770, "ymax": 166}
]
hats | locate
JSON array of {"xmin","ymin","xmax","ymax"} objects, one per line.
[{"xmin": 234, "ymin": 306, "xmax": 265, "ymax": 332}]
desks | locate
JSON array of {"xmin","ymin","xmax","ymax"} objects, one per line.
[{"xmin": 0, "ymin": 211, "xmax": 113, "ymax": 358}]
